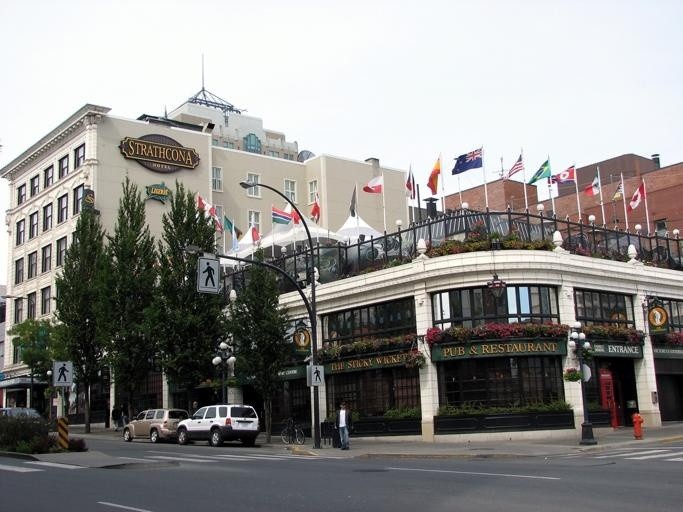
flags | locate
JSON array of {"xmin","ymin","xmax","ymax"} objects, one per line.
[
  {"xmin": 627, "ymin": 180, "xmax": 644, "ymax": 213},
  {"xmin": 558, "ymin": 165, "xmax": 575, "ymax": 183},
  {"xmin": 361, "ymin": 175, "xmax": 382, "ymax": 195},
  {"xmin": 527, "ymin": 160, "xmax": 551, "ymax": 186},
  {"xmin": 583, "ymin": 167, "xmax": 600, "ymax": 197},
  {"xmin": 349, "ymin": 187, "xmax": 356, "ymax": 217},
  {"xmin": 196, "ymin": 193, "xmax": 321, "ymax": 251},
  {"xmin": 404, "ymin": 164, "xmax": 417, "ymax": 200},
  {"xmin": 547, "ymin": 175, "xmax": 558, "ymax": 186},
  {"xmin": 450, "ymin": 146, "xmax": 482, "ymax": 175},
  {"xmin": 425, "ymin": 157, "xmax": 440, "ymax": 196},
  {"xmin": 507, "ymin": 153, "xmax": 523, "ymax": 178},
  {"xmin": 611, "ymin": 179, "xmax": 624, "ymax": 202}
]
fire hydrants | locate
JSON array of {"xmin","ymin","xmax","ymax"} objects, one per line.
[{"xmin": 633, "ymin": 412, "xmax": 644, "ymax": 439}]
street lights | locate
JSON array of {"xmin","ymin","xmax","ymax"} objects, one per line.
[
  {"xmin": 395, "ymin": 219, "xmax": 403, "ymax": 260},
  {"xmin": 461, "ymin": 202, "xmax": 469, "ymax": 238},
  {"xmin": 211, "ymin": 341, "xmax": 237, "ymax": 404},
  {"xmin": 570, "ymin": 320, "xmax": 598, "ymax": 445},
  {"xmin": 1, "ymin": 294, "xmax": 34, "ymax": 404},
  {"xmin": 45, "ymin": 369, "xmax": 54, "ymax": 421},
  {"xmin": 634, "ymin": 224, "xmax": 642, "ymax": 257},
  {"xmin": 672, "ymin": 228, "xmax": 682, "ymax": 266},
  {"xmin": 588, "ymin": 215, "xmax": 596, "ymax": 253},
  {"xmin": 536, "ymin": 203, "xmax": 546, "ymax": 241},
  {"xmin": 279, "ymin": 245, "xmax": 286, "ymax": 271},
  {"xmin": 238, "ymin": 180, "xmax": 320, "ymax": 449}
]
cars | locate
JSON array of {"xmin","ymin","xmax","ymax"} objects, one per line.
[{"xmin": 0, "ymin": 407, "xmax": 41, "ymax": 423}]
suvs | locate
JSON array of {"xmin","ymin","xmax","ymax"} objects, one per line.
[
  {"xmin": 122, "ymin": 407, "xmax": 188, "ymax": 444},
  {"xmin": 175, "ymin": 404, "xmax": 260, "ymax": 446}
]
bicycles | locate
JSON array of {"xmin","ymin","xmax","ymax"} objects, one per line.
[{"xmin": 280, "ymin": 417, "xmax": 304, "ymax": 445}]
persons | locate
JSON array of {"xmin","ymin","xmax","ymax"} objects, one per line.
[
  {"xmin": 118, "ymin": 403, "xmax": 127, "ymax": 430},
  {"xmin": 332, "ymin": 400, "xmax": 354, "ymax": 450},
  {"xmin": 110, "ymin": 405, "xmax": 119, "ymax": 433},
  {"xmin": 187, "ymin": 401, "xmax": 198, "ymax": 419}
]
